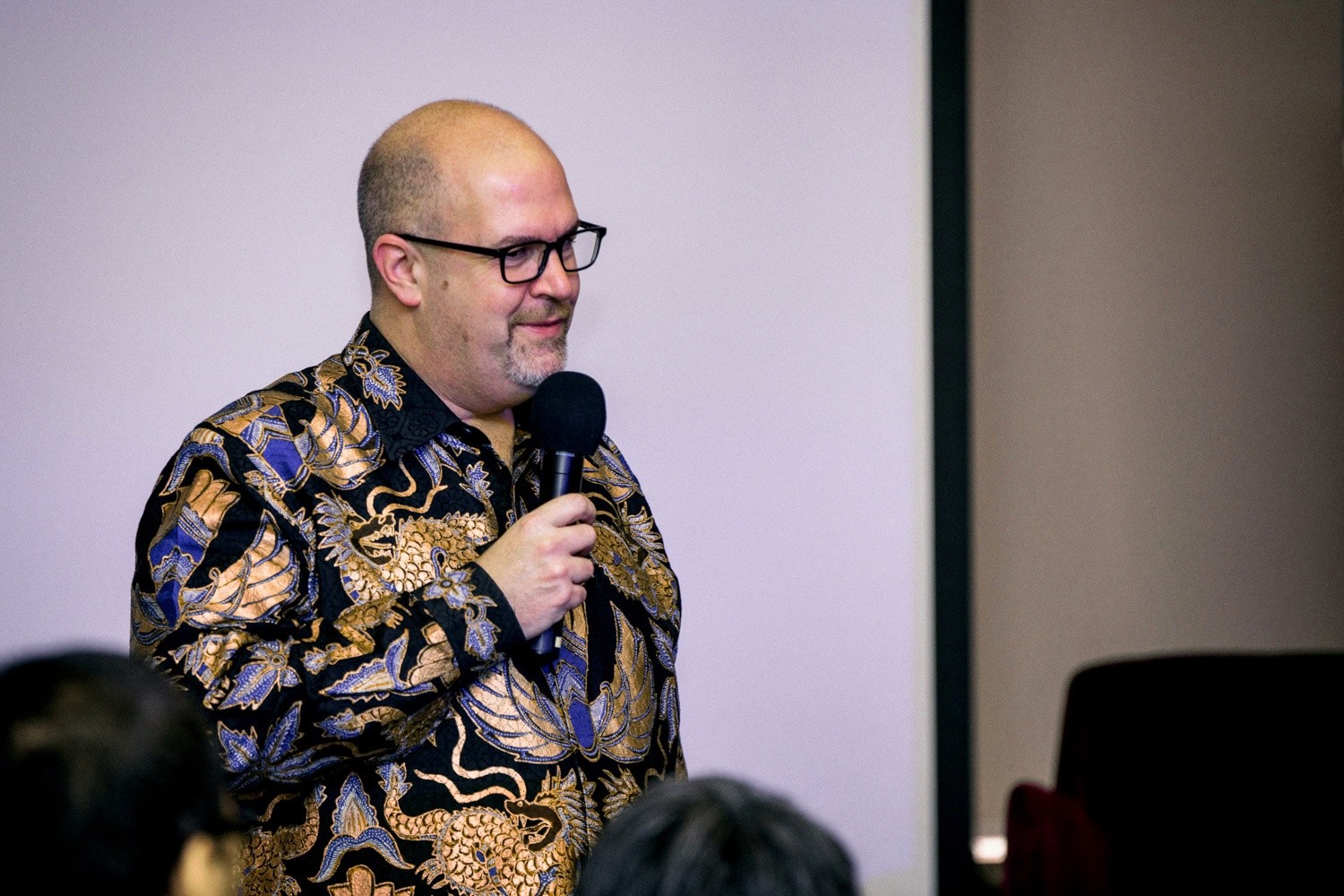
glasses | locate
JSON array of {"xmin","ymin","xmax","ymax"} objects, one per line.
[{"xmin": 393, "ymin": 220, "xmax": 607, "ymax": 285}]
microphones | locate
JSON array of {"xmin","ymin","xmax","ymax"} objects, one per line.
[{"xmin": 530, "ymin": 371, "xmax": 607, "ymax": 663}]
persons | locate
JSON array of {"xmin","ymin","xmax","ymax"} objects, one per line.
[
  {"xmin": 0, "ymin": 645, "xmax": 243, "ymax": 896},
  {"xmin": 574, "ymin": 768, "xmax": 864, "ymax": 895},
  {"xmin": 129, "ymin": 96, "xmax": 689, "ymax": 896}
]
